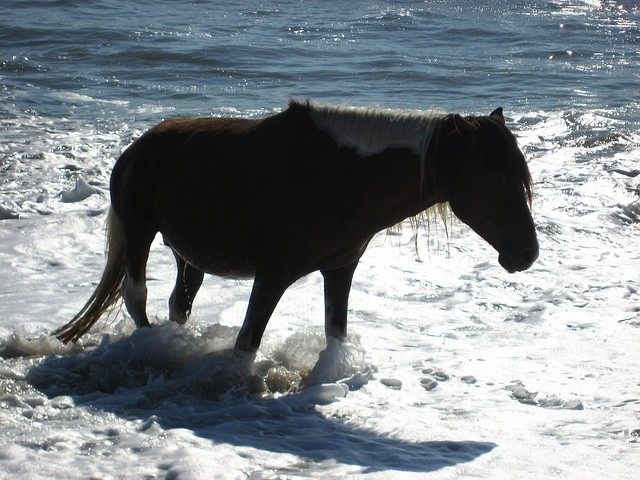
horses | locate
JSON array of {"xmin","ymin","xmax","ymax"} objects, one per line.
[{"xmin": 49, "ymin": 98, "xmax": 538, "ymax": 358}]
